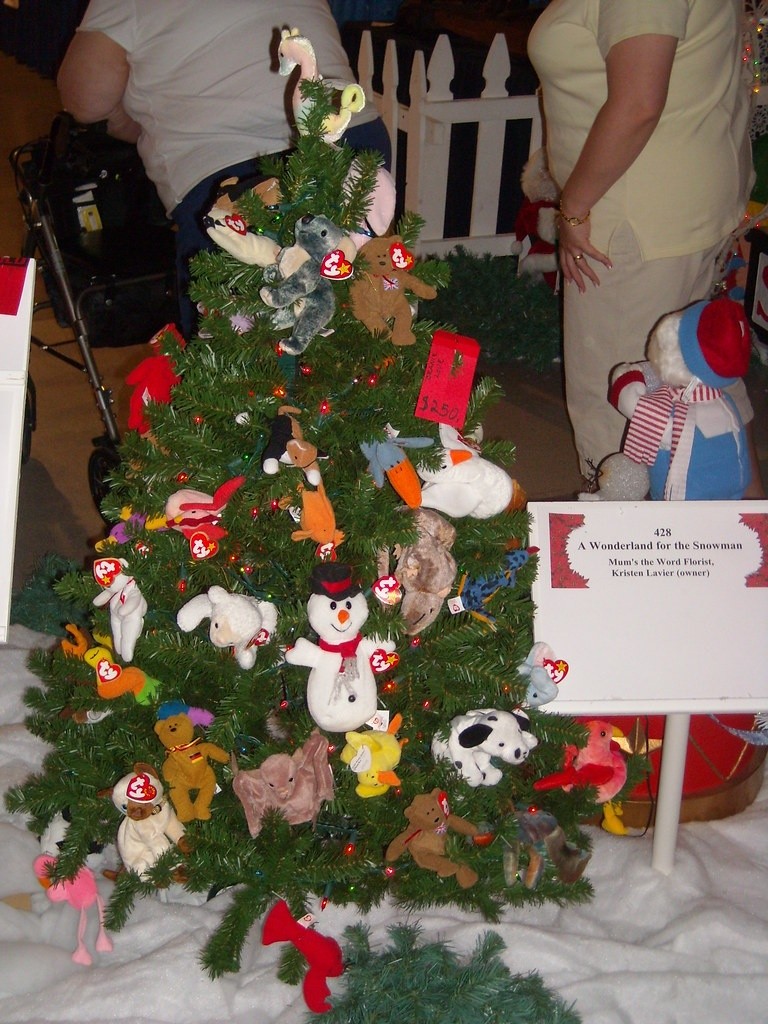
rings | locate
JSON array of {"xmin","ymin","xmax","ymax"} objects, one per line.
[{"xmin": 574, "ymin": 254, "xmax": 583, "ymax": 261}]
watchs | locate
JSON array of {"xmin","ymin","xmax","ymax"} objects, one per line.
[{"xmin": 559, "ymin": 193, "xmax": 590, "ymax": 226}]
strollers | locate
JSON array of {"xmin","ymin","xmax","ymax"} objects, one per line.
[{"xmin": 9, "ymin": 110, "xmax": 179, "ymax": 529}]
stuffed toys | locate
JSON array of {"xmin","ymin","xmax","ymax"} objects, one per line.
[
  {"xmin": 32, "ymin": 28, "xmax": 637, "ymax": 1013},
  {"xmin": 580, "ymin": 298, "xmax": 751, "ymax": 501}
]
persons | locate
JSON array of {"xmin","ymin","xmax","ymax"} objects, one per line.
[
  {"xmin": 58, "ymin": 0, "xmax": 392, "ymax": 350},
  {"xmin": 527, "ymin": 0, "xmax": 752, "ymax": 486}
]
